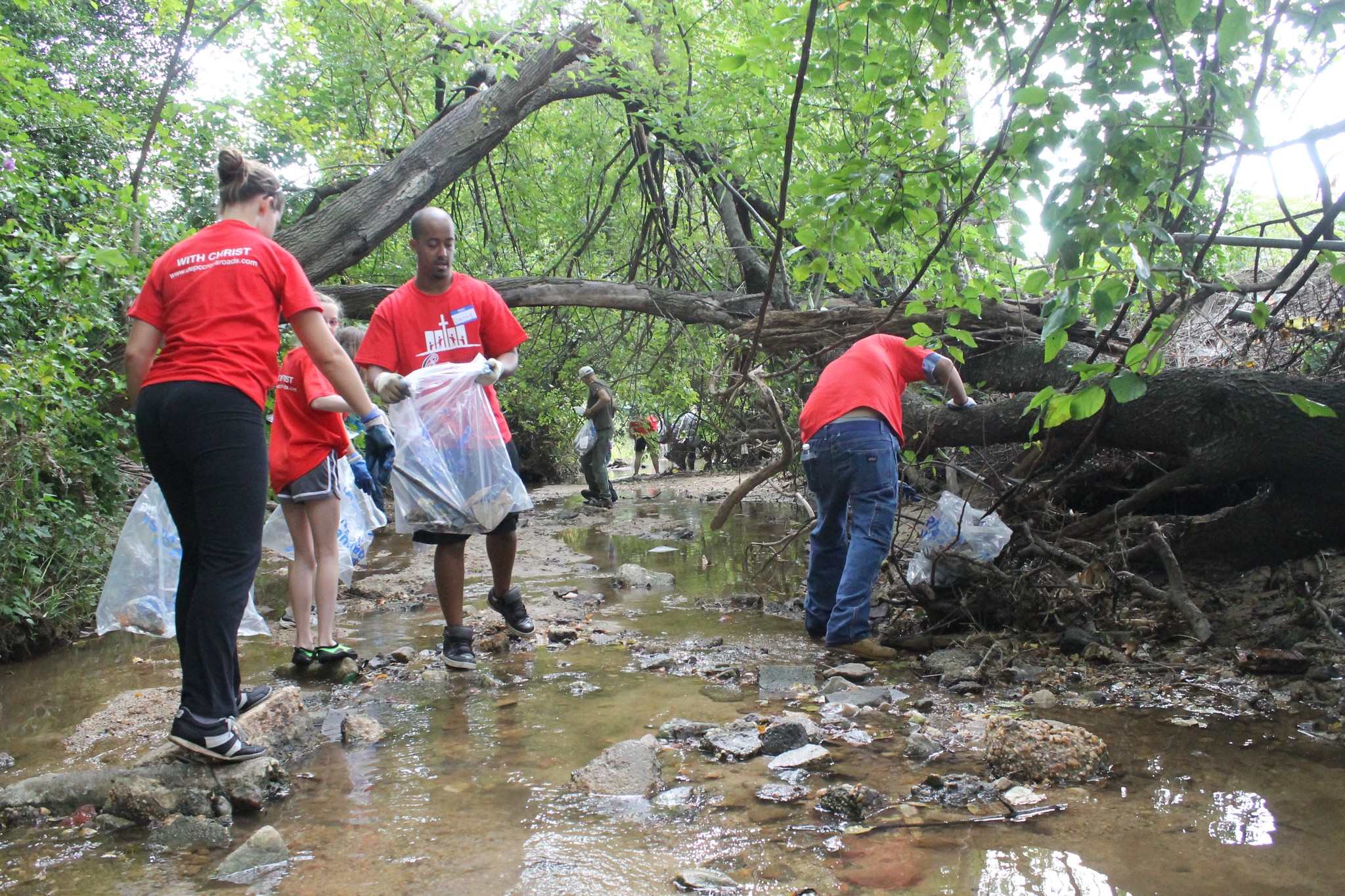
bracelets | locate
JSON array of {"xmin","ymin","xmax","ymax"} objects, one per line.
[{"xmin": 361, "ymin": 409, "xmax": 380, "ymax": 423}]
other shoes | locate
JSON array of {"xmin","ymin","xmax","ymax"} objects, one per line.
[
  {"xmin": 583, "ymin": 499, "xmax": 597, "ymax": 505},
  {"xmin": 826, "ymin": 636, "xmax": 897, "ymax": 663},
  {"xmin": 581, "ymin": 490, "xmax": 597, "ymax": 500}
]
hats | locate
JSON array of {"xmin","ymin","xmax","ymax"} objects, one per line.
[{"xmin": 579, "ymin": 366, "xmax": 594, "ymax": 378}]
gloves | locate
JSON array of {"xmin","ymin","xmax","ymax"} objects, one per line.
[
  {"xmin": 946, "ymin": 395, "xmax": 977, "ymax": 409},
  {"xmin": 348, "ymin": 452, "xmax": 376, "ymax": 496},
  {"xmin": 361, "ymin": 414, "xmax": 396, "ymax": 485},
  {"xmin": 583, "ymin": 409, "xmax": 595, "ymax": 419},
  {"xmin": 474, "ymin": 357, "xmax": 505, "ymax": 386},
  {"xmin": 372, "ymin": 371, "xmax": 413, "ymax": 405},
  {"xmin": 893, "ymin": 479, "xmax": 921, "ymax": 504}
]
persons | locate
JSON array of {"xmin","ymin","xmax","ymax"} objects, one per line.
[
  {"xmin": 120, "ymin": 146, "xmax": 398, "ymax": 764},
  {"xmin": 798, "ymin": 335, "xmax": 979, "ymax": 664},
  {"xmin": 352, "ymin": 207, "xmax": 534, "ymax": 668},
  {"xmin": 269, "ymin": 292, "xmax": 698, "ymax": 669}
]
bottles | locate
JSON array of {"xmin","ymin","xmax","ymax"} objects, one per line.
[{"xmin": 801, "ymin": 445, "xmax": 815, "ymax": 460}]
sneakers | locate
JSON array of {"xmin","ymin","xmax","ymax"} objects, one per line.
[
  {"xmin": 235, "ymin": 685, "xmax": 273, "ymax": 715},
  {"xmin": 440, "ymin": 626, "xmax": 477, "ymax": 669},
  {"xmin": 314, "ymin": 640, "xmax": 358, "ymax": 663},
  {"xmin": 291, "ymin": 645, "xmax": 318, "ymax": 665},
  {"xmin": 168, "ymin": 705, "xmax": 268, "ymax": 762},
  {"xmin": 279, "ymin": 607, "xmax": 319, "ymax": 628},
  {"xmin": 486, "ymin": 586, "xmax": 536, "ymax": 638},
  {"xmin": 312, "ymin": 602, "xmax": 347, "ymax": 616}
]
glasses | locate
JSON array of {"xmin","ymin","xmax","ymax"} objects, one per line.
[{"xmin": 324, "ymin": 319, "xmax": 342, "ymax": 329}]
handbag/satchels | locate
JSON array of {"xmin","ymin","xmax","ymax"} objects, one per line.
[
  {"xmin": 907, "ymin": 491, "xmax": 1014, "ymax": 584},
  {"xmin": 389, "ymin": 361, "xmax": 534, "ymax": 536},
  {"xmin": 96, "ymin": 479, "xmax": 273, "ymax": 639},
  {"xmin": 573, "ymin": 418, "xmax": 598, "ymax": 457},
  {"xmin": 262, "ymin": 454, "xmax": 388, "ymax": 591}
]
